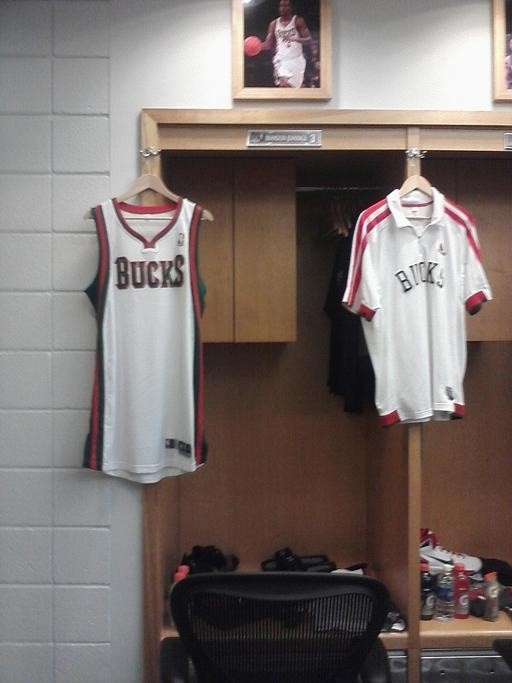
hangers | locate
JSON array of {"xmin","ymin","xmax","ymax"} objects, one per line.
[
  {"xmin": 85, "ymin": 152, "xmax": 213, "ymax": 220},
  {"xmin": 353, "ymin": 147, "xmax": 475, "ymax": 237},
  {"xmin": 316, "ymin": 181, "xmax": 384, "ymax": 241}
]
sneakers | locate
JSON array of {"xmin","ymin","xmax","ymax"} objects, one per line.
[
  {"xmin": 419, "ymin": 528, "xmax": 483, "ymax": 574},
  {"xmin": 182, "ymin": 544, "xmax": 238, "ymax": 574}
]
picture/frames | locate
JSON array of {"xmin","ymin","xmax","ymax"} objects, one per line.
[
  {"xmin": 231, "ymin": 0, "xmax": 333, "ymax": 101},
  {"xmin": 491, "ymin": 0, "xmax": 511, "ymax": 101}
]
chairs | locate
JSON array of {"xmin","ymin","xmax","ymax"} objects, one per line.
[{"xmin": 161, "ymin": 572, "xmax": 390, "ymax": 680}]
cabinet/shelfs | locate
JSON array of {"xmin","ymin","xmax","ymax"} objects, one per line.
[{"xmin": 140, "ymin": 105, "xmax": 511, "ymax": 680}]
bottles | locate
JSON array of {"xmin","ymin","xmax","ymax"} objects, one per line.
[{"xmin": 421, "ymin": 563, "xmax": 499, "ymax": 623}]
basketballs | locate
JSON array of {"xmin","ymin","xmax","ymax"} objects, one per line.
[{"xmin": 244, "ymin": 36, "xmax": 261, "ymax": 56}]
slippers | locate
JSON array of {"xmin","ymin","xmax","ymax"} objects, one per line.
[{"xmin": 261, "ymin": 546, "xmax": 336, "ymax": 572}]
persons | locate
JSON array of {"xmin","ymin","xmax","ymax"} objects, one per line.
[
  {"xmin": 256, "ymin": 0, "xmax": 312, "ymax": 89},
  {"xmin": 303, "ymin": 30, "xmax": 321, "ymax": 88},
  {"xmin": 505, "ymin": 37, "xmax": 512, "ymax": 88}
]
